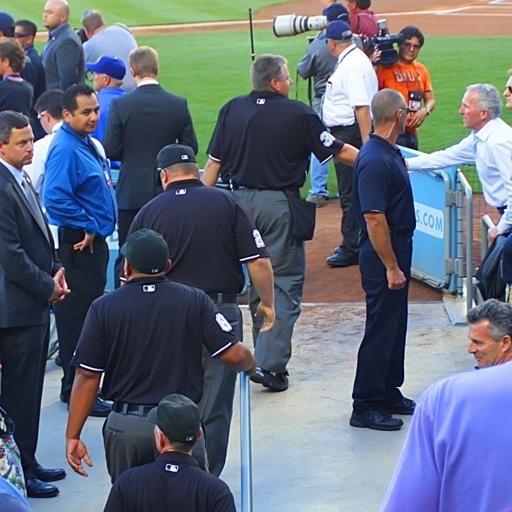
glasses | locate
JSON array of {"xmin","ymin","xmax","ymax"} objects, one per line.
[{"xmin": 405, "ymin": 43, "xmax": 421, "ymax": 49}]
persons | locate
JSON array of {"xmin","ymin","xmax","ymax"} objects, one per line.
[
  {"xmin": 298, "ymin": 0, "xmax": 436, "ymax": 268},
  {"xmin": 405, "ymin": 68, "xmax": 512, "ymax": 302},
  {"xmin": 382, "ymin": 298, "xmax": 511, "ymax": 512},
  {"xmin": 350, "ymin": 89, "xmax": 416, "ymax": 429},
  {"xmin": 205, "ymin": 53, "xmax": 359, "ymax": 389},
  {"xmin": 0, "ymin": 0, "xmax": 275, "ymax": 512}
]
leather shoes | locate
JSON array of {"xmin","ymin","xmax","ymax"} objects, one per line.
[
  {"xmin": 91, "ymin": 401, "xmax": 113, "ymax": 417},
  {"xmin": 27, "ymin": 466, "xmax": 66, "ymax": 499},
  {"xmin": 325, "ymin": 244, "xmax": 362, "ymax": 269}
]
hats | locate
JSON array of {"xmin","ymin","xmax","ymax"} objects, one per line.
[
  {"xmin": 85, "ymin": 53, "xmax": 126, "ymax": 79},
  {"xmin": 154, "ymin": 140, "xmax": 197, "ymax": 189},
  {"xmin": 316, "ymin": 3, "xmax": 352, "ymax": 42},
  {"xmin": 148, "ymin": 392, "xmax": 202, "ymax": 442},
  {"xmin": 0, "ymin": 10, "xmax": 15, "ymax": 32},
  {"xmin": 119, "ymin": 225, "xmax": 170, "ymax": 274}
]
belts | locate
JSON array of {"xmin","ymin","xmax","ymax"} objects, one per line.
[{"xmin": 114, "ymin": 403, "xmax": 151, "ymax": 415}]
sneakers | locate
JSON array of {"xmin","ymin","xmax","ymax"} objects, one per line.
[
  {"xmin": 349, "ymin": 401, "xmax": 405, "ymax": 431},
  {"xmin": 303, "ymin": 191, "xmax": 330, "ymax": 207},
  {"xmin": 386, "ymin": 398, "xmax": 416, "ymax": 416},
  {"xmin": 251, "ymin": 363, "xmax": 289, "ymax": 392}
]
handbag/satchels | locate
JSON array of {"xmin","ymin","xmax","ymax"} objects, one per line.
[{"xmin": 283, "ymin": 191, "xmax": 318, "ymax": 242}]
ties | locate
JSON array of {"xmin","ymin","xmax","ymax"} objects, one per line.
[{"xmin": 21, "ymin": 177, "xmax": 50, "ymax": 245}]
